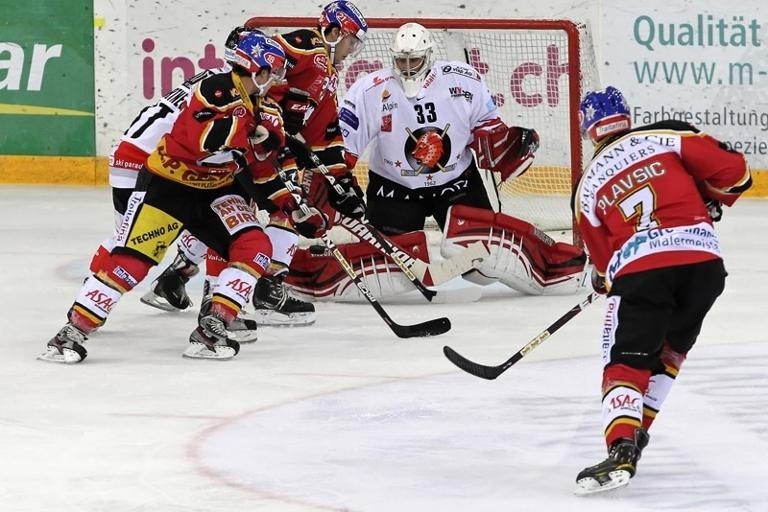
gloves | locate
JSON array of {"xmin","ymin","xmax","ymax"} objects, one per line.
[
  {"xmin": 291, "ymin": 206, "xmax": 329, "ymax": 238},
  {"xmin": 591, "ymin": 267, "xmax": 608, "ymax": 293},
  {"xmin": 254, "ymin": 125, "xmax": 283, "ymax": 154},
  {"xmin": 705, "ymin": 197, "xmax": 723, "ymax": 221},
  {"xmin": 328, "ymin": 176, "xmax": 361, "ymax": 213}
]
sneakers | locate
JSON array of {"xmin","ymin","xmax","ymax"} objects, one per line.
[
  {"xmin": 189, "ymin": 314, "xmax": 240, "ymax": 356},
  {"xmin": 576, "ymin": 428, "xmax": 649, "ymax": 485},
  {"xmin": 253, "ymin": 268, "xmax": 315, "ymax": 313},
  {"xmin": 48, "ymin": 322, "xmax": 87, "ymax": 361}
]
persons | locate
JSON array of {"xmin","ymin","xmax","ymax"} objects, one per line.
[
  {"xmin": 109, "ymin": 27, "xmax": 316, "ymax": 317},
  {"xmin": 197, "ymin": 1, "xmax": 371, "ymax": 341},
  {"xmin": 336, "ymin": 19, "xmax": 541, "ymax": 284},
  {"xmin": 571, "ymin": 85, "xmax": 752, "ymax": 493},
  {"xmin": 44, "ymin": 34, "xmax": 332, "ymax": 362}
]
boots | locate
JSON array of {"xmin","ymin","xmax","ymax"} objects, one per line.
[
  {"xmin": 151, "ymin": 246, "xmax": 200, "ymax": 309},
  {"xmin": 200, "ymin": 279, "xmax": 257, "ymax": 331}
]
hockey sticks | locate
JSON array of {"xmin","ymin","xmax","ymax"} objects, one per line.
[
  {"xmin": 271, "ymin": 157, "xmax": 452, "ymax": 337},
  {"xmin": 442, "ymin": 290, "xmax": 603, "ymax": 378},
  {"xmin": 293, "ymin": 131, "xmax": 484, "ymax": 303}
]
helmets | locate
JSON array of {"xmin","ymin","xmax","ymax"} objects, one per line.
[
  {"xmin": 236, "ymin": 32, "xmax": 285, "ymax": 72},
  {"xmin": 389, "ymin": 22, "xmax": 435, "ymax": 98},
  {"xmin": 579, "ymin": 87, "xmax": 631, "ymax": 145},
  {"xmin": 319, "ymin": 0, "xmax": 368, "ymax": 42},
  {"xmin": 225, "ymin": 27, "xmax": 266, "ymax": 61}
]
